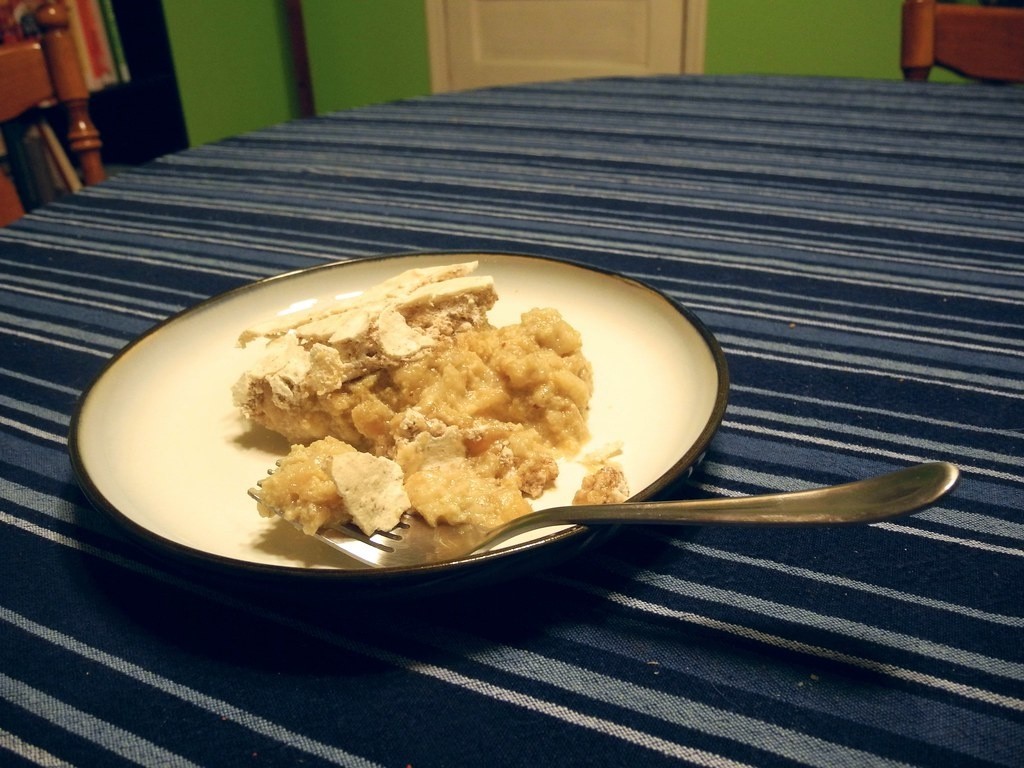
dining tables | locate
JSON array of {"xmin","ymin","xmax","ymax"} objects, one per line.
[{"xmin": 0, "ymin": 72, "xmax": 1024, "ymax": 768}]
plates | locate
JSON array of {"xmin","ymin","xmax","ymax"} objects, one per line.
[{"xmin": 69, "ymin": 249, "xmax": 731, "ymax": 591}]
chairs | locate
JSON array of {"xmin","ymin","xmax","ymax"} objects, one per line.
[
  {"xmin": 0, "ymin": 0, "xmax": 107, "ymax": 224},
  {"xmin": 899, "ymin": 0, "xmax": 1024, "ymax": 82}
]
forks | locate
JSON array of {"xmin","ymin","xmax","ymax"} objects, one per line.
[{"xmin": 247, "ymin": 456, "xmax": 961, "ymax": 566}]
